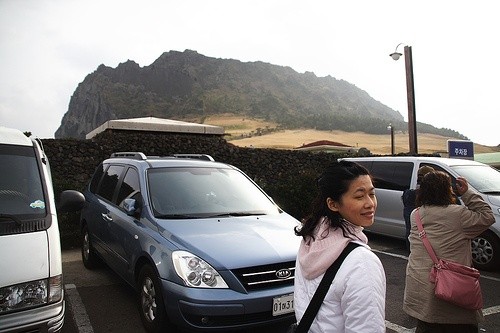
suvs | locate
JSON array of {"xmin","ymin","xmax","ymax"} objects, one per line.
[{"xmin": 82, "ymin": 151, "xmax": 305, "ymax": 333}]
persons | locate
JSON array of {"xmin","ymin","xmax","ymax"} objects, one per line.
[
  {"xmin": 293, "ymin": 160, "xmax": 386, "ymax": 333},
  {"xmin": 402, "ymin": 171, "xmax": 496, "ymax": 333},
  {"xmin": 402, "ymin": 165, "xmax": 462, "ymax": 258}
]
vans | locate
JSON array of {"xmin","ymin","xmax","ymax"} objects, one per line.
[
  {"xmin": 337, "ymin": 156, "xmax": 496, "ymax": 269},
  {"xmin": 0, "ymin": 126, "xmax": 87, "ymax": 332}
]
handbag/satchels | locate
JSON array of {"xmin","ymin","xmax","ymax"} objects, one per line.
[{"xmin": 415, "ymin": 207, "xmax": 483, "ymax": 310}]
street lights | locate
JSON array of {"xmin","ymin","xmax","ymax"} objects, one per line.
[
  {"xmin": 390, "ymin": 42, "xmax": 418, "ymax": 153},
  {"xmin": 388, "ymin": 122, "xmax": 394, "ymax": 155}
]
